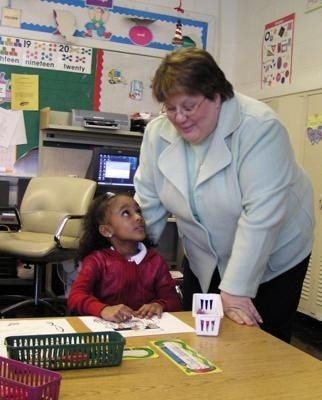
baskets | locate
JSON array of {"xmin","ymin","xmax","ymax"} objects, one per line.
[
  {"xmin": 191, "ymin": 293, "xmax": 224, "ymax": 337},
  {"xmin": 4, "ymin": 331, "xmax": 126, "ymax": 375},
  {"xmin": 0, "ymin": 356, "xmax": 62, "ymax": 399}
]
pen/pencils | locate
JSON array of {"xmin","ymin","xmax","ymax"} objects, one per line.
[{"xmin": 21, "ymin": 351, "xmax": 112, "ymax": 370}]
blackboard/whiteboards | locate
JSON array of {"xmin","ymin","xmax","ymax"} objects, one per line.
[{"xmin": 0, "ymin": 36, "xmax": 98, "ymax": 185}]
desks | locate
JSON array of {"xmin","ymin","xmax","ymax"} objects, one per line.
[{"xmin": 1, "ymin": 308, "xmax": 322, "ymax": 400}]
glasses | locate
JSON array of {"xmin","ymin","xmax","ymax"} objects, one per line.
[{"xmin": 160, "ymin": 96, "xmax": 206, "ymax": 119}]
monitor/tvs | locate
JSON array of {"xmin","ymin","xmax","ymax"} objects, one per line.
[{"xmin": 97, "ymin": 149, "xmax": 140, "ymax": 189}]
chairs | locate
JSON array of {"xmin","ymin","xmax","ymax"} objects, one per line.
[{"xmin": 1, "ymin": 174, "xmax": 99, "ymax": 317}]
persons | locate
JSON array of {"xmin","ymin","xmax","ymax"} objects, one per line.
[
  {"xmin": 67, "ymin": 192, "xmax": 180, "ymax": 323},
  {"xmin": 133, "ymin": 47, "xmax": 315, "ymax": 343}
]
